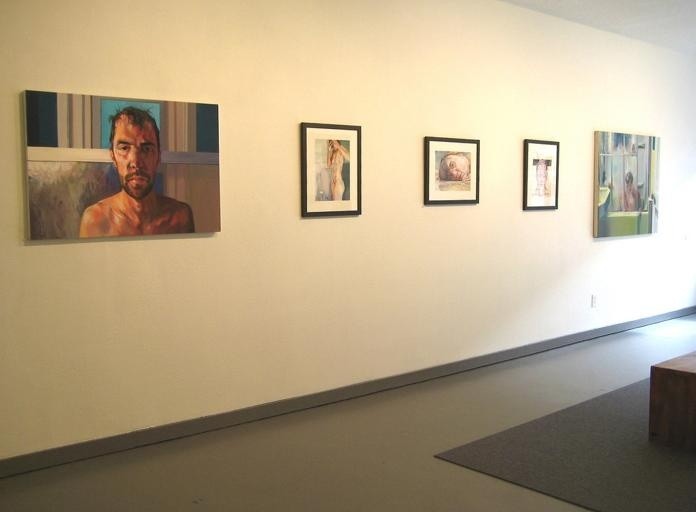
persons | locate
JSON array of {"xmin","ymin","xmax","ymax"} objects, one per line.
[
  {"xmin": 439, "ymin": 152, "xmax": 473, "ymax": 186},
  {"xmin": 530, "ymin": 159, "xmax": 551, "ymax": 199},
  {"xmin": 620, "ymin": 172, "xmax": 642, "ymax": 212},
  {"xmin": 326, "ymin": 140, "xmax": 349, "ymax": 202},
  {"xmin": 75, "ymin": 105, "xmax": 195, "ymax": 238}
]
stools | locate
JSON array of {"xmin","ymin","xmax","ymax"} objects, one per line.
[{"xmin": 650, "ymin": 352, "xmax": 696, "ymax": 443}]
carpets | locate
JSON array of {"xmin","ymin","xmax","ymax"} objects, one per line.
[{"xmin": 431, "ymin": 353, "xmax": 696, "ymax": 512}]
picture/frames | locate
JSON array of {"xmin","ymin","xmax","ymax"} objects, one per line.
[
  {"xmin": 425, "ymin": 137, "xmax": 480, "ymax": 205},
  {"xmin": 301, "ymin": 123, "xmax": 361, "ymax": 217},
  {"xmin": 523, "ymin": 140, "xmax": 560, "ymax": 212}
]
